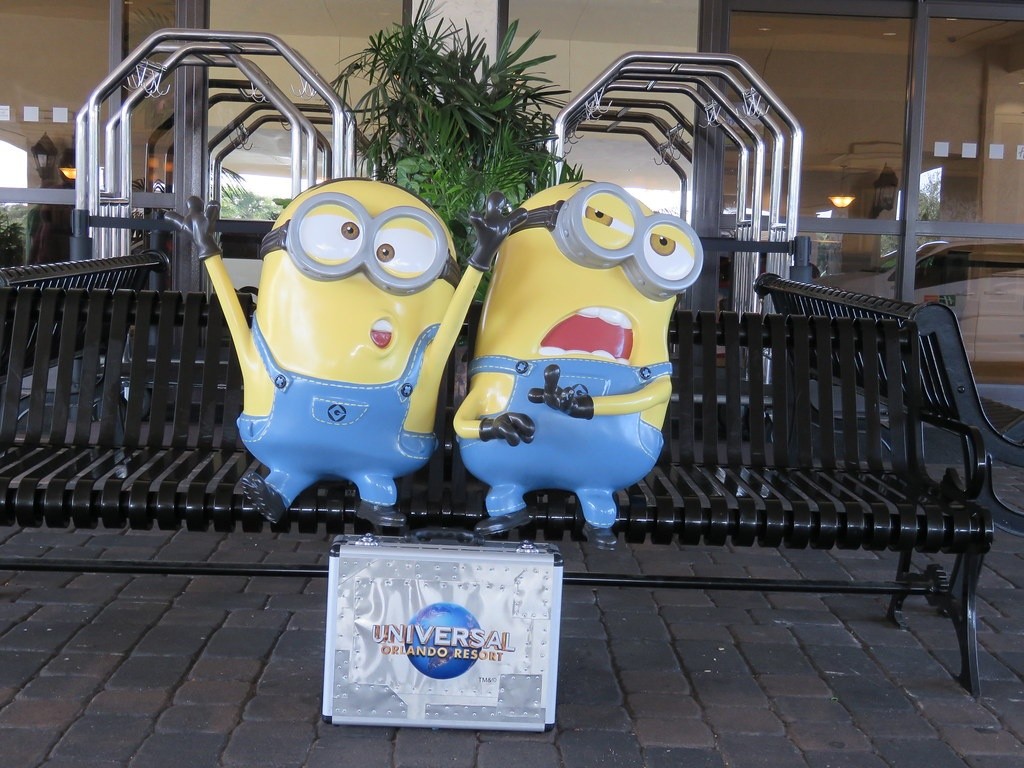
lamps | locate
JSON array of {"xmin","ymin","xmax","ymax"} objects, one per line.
[
  {"xmin": 31, "ymin": 130, "xmax": 57, "ymax": 180},
  {"xmin": 58, "ymin": 128, "xmax": 76, "ymax": 180},
  {"xmin": 827, "ymin": 165, "xmax": 856, "ymax": 208}
]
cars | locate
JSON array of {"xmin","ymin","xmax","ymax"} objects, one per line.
[{"xmin": 814, "ymin": 237, "xmax": 1024, "ymax": 364}]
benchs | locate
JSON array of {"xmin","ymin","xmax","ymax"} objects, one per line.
[{"xmin": 0, "ymin": 249, "xmax": 1024, "ymax": 698}]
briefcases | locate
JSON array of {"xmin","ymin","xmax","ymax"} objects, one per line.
[{"xmin": 321, "ymin": 525, "xmax": 566, "ymax": 734}]
